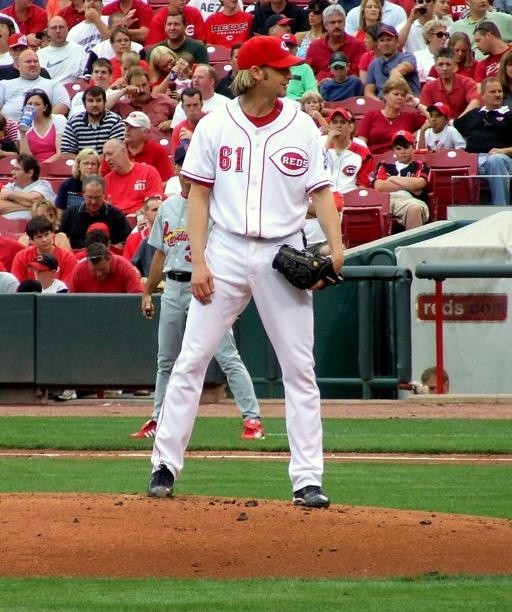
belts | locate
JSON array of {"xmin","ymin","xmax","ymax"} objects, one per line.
[{"xmin": 167, "ymin": 271, "xmax": 191, "ymax": 282}]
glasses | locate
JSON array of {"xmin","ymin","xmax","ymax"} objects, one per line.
[
  {"xmin": 432, "ymin": 31, "xmax": 451, "ymax": 38},
  {"xmin": 25, "ymin": 90, "xmax": 42, "ymax": 93},
  {"xmin": 307, "ymin": 9, "xmax": 321, "ymax": 15}
]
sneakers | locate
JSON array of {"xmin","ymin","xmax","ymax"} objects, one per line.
[
  {"xmin": 149, "ymin": 469, "xmax": 174, "ymax": 497},
  {"xmin": 53, "ymin": 389, "xmax": 77, "ymax": 401},
  {"xmin": 129, "ymin": 420, "xmax": 158, "ymax": 437},
  {"xmin": 242, "ymin": 419, "xmax": 263, "ymax": 439},
  {"xmin": 293, "ymin": 486, "xmax": 329, "ymax": 507}
]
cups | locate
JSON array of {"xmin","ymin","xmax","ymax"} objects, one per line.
[{"xmin": 17, "ymin": 104, "xmax": 35, "ymax": 131}]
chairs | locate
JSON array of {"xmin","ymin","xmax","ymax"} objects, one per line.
[{"xmin": 1, "ymin": 1, "xmax": 482, "ymax": 295}]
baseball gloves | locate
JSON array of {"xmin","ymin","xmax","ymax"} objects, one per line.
[{"xmin": 272, "ymin": 244, "xmax": 344, "ymax": 291}]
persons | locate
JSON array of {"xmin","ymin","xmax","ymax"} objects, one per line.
[
  {"xmin": 147, "ymin": 36, "xmax": 343, "ymax": 506},
  {"xmin": 129, "ymin": 143, "xmax": 265, "ymax": 440},
  {"xmin": 1, "ymin": 0, "xmax": 511, "ymax": 400}
]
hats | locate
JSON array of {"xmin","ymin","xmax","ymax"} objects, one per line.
[
  {"xmin": 237, "ymin": 36, "xmax": 306, "ymax": 70},
  {"xmin": 376, "ymin": 26, "xmax": 399, "ymax": 38},
  {"xmin": 265, "ymin": 14, "xmax": 294, "ymax": 28},
  {"xmin": 7, "ymin": 34, "xmax": 27, "ymax": 47},
  {"xmin": 330, "ymin": 107, "xmax": 351, "ymax": 122},
  {"xmin": 427, "ymin": 103, "xmax": 451, "ymax": 120},
  {"xmin": 392, "ymin": 130, "xmax": 415, "ymax": 144},
  {"xmin": 30, "ymin": 254, "xmax": 58, "ymax": 271},
  {"xmin": 281, "ymin": 33, "xmax": 299, "ymax": 45},
  {"xmin": 122, "ymin": 111, "xmax": 150, "ymax": 131},
  {"xmin": 87, "ymin": 223, "xmax": 110, "ymax": 238},
  {"xmin": 35, "ymin": 28, "xmax": 49, "ymax": 38},
  {"xmin": 174, "ymin": 145, "xmax": 189, "ymax": 163},
  {"xmin": 329, "ymin": 52, "xmax": 348, "ymax": 67}
]
